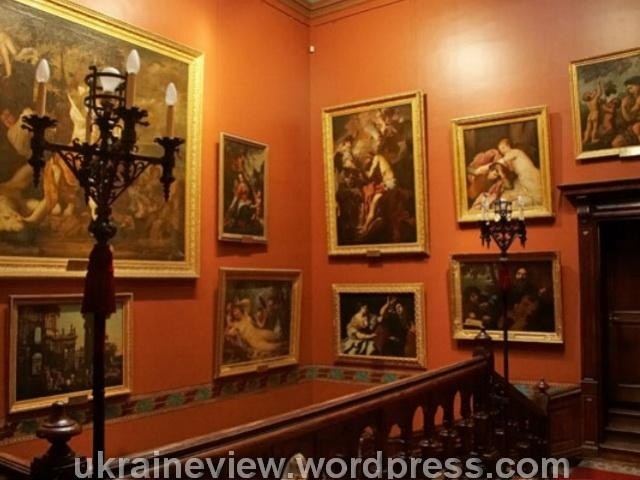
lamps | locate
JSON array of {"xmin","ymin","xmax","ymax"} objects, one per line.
[{"xmin": 20, "ymin": 48, "xmax": 186, "ymax": 480}]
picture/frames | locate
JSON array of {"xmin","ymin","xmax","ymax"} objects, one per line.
[{"xmin": 0, "ymin": 0, "xmax": 640, "ymax": 415}]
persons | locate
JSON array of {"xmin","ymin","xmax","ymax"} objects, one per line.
[
  {"xmin": 580, "ymin": 76, "xmax": 603, "ymax": 144},
  {"xmin": 467, "ymin": 148, "xmax": 517, "ymax": 203},
  {"xmin": 332, "ymin": 106, "xmax": 418, "ymax": 246},
  {"xmin": 494, "ymin": 265, "xmax": 549, "ymax": 331},
  {"xmin": 611, "ymin": 75, "xmax": 640, "ymax": 147},
  {"xmin": 0, "ymin": 12, "xmax": 181, "ymax": 250},
  {"xmin": 374, "ymin": 296, "xmax": 409, "ymax": 355},
  {"xmin": 227, "ymin": 146, "xmax": 265, "ymax": 229},
  {"xmin": 462, "ymin": 286, "xmax": 503, "ymax": 330},
  {"xmin": 223, "ymin": 280, "xmax": 292, "ymax": 360},
  {"xmin": 601, "ymin": 86, "xmax": 621, "ymax": 136},
  {"xmin": 466, "ymin": 138, "xmax": 543, "ymax": 209},
  {"xmin": 339, "ymin": 295, "xmax": 383, "ymax": 356}
]
